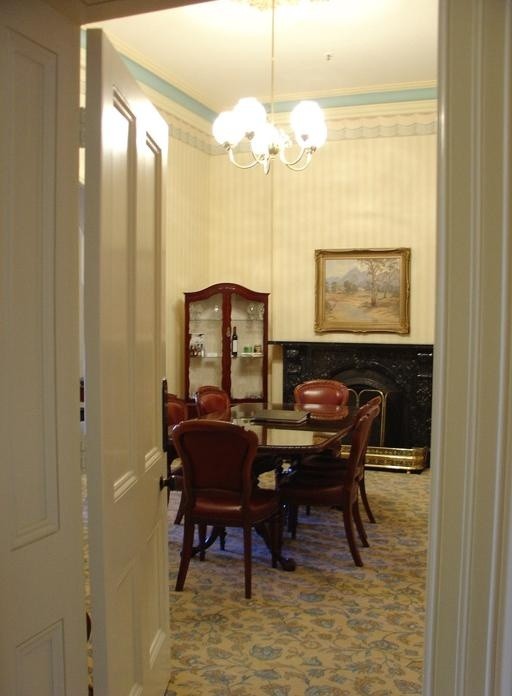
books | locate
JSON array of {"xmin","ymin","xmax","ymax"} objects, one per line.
[{"xmin": 254, "ymin": 410, "xmax": 310, "ymax": 424}]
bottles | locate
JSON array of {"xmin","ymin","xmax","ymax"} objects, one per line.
[
  {"xmin": 232, "ymin": 326, "xmax": 238, "ymax": 357},
  {"xmin": 244, "ymin": 344, "xmax": 261, "ymax": 354}
]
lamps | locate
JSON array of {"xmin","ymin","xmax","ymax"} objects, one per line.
[{"xmin": 210, "ymin": 0, "xmax": 327, "ymax": 177}]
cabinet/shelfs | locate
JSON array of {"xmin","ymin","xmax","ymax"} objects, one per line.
[{"xmin": 179, "ymin": 283, "xmax": 272, "ymax": 411}]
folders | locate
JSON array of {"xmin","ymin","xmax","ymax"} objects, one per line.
[{"xmin": 250, "ymin": 409, "xmax": 310, "ymax": 425}]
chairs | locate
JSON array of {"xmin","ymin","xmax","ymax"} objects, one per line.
[{"xmin": 163, "ymin": 377, "xmax": 386, "ymax": 604}]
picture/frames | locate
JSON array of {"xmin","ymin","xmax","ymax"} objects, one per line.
[{"xmin": 312, "ymin": 246, "xmax": 418, "ymax": 338}]
